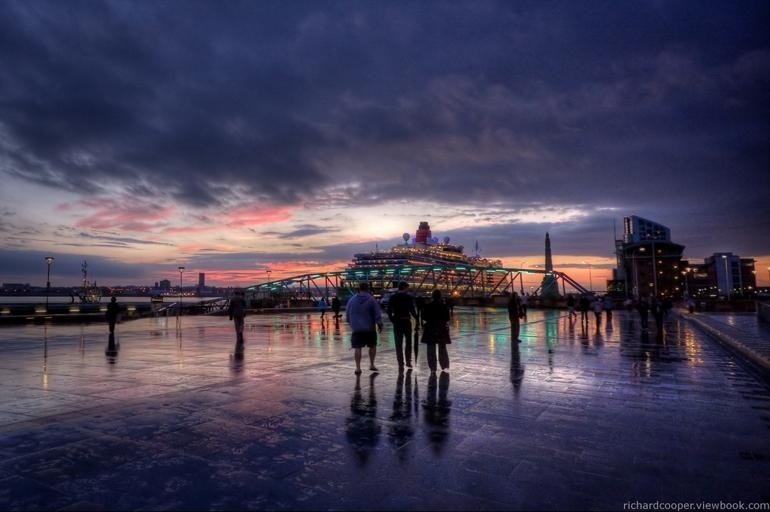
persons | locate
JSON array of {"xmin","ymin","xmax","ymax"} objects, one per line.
[
  {"xmin": 104, "ymin": 296, "xmax": 121, "ymax": 332},
  {"xmin": 508, "ymin": 291, "xmax": 524, "ymax": 343},
  {"xmin": 591, "ymin": 298, "xmax": 602, "ymax": 325},
  {"xmin": 387, "ymin": 281, "xmax": 420, "ymax": 368},
  {"xmin": 580, "ymin": 294, "xmax": 589, "ymax": 322},
  {"xmin": 623, "ymin": 286, "xmax": 694, "ymax": 325},
  {"xmin": 520, "ymin": 291, "xmax": 527, "ymax": 315},
  {"xmin": 567, "ymin": 294, "xmax": 577, "ymax": 318},
  {"xmin": 227, "ymin": 288, "xmax": 246, "ymax": 338},
  {"xmin": 346, "ymin": 281, "xmax": 383, "ymax": 374},
  {"xmin": 320, "ymin": 296, "xmax": 326, "ymax": 317},
  {"xmin": 604, "ymin": 297, "xmax": 614, "ymax": 321},
  {"xmin": 332, "ymin": 294, "xmax": 340, "ymax": 319},
  {"xmin": 420, "ymin": 289, "xmax": 452, "ymax": 373}
]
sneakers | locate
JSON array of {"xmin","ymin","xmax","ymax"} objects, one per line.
[{"xmin": 399, "ymin": 362, "xmax": 412, "ymax": 367}]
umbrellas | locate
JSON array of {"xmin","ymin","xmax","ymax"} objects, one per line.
[{"xmin": 413, "ymin": 321, "xmax": 420, "ymax": 365}]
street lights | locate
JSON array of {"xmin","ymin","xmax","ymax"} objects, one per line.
[
  {"xmin": 43, "ymin": 255, "xmax": 55, "ymax": 287},
  {"xmin": 721, "ymin": 254, "xmax": 731, "ymax": 301},
  {"xmin": 178, "ymin": 265, "xmax": 185, "ymax": 296}
]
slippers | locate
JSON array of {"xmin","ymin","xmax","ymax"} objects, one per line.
[{"xmin": 354, "ymin": 367, "xmax": 378, "ymax": 374}]
